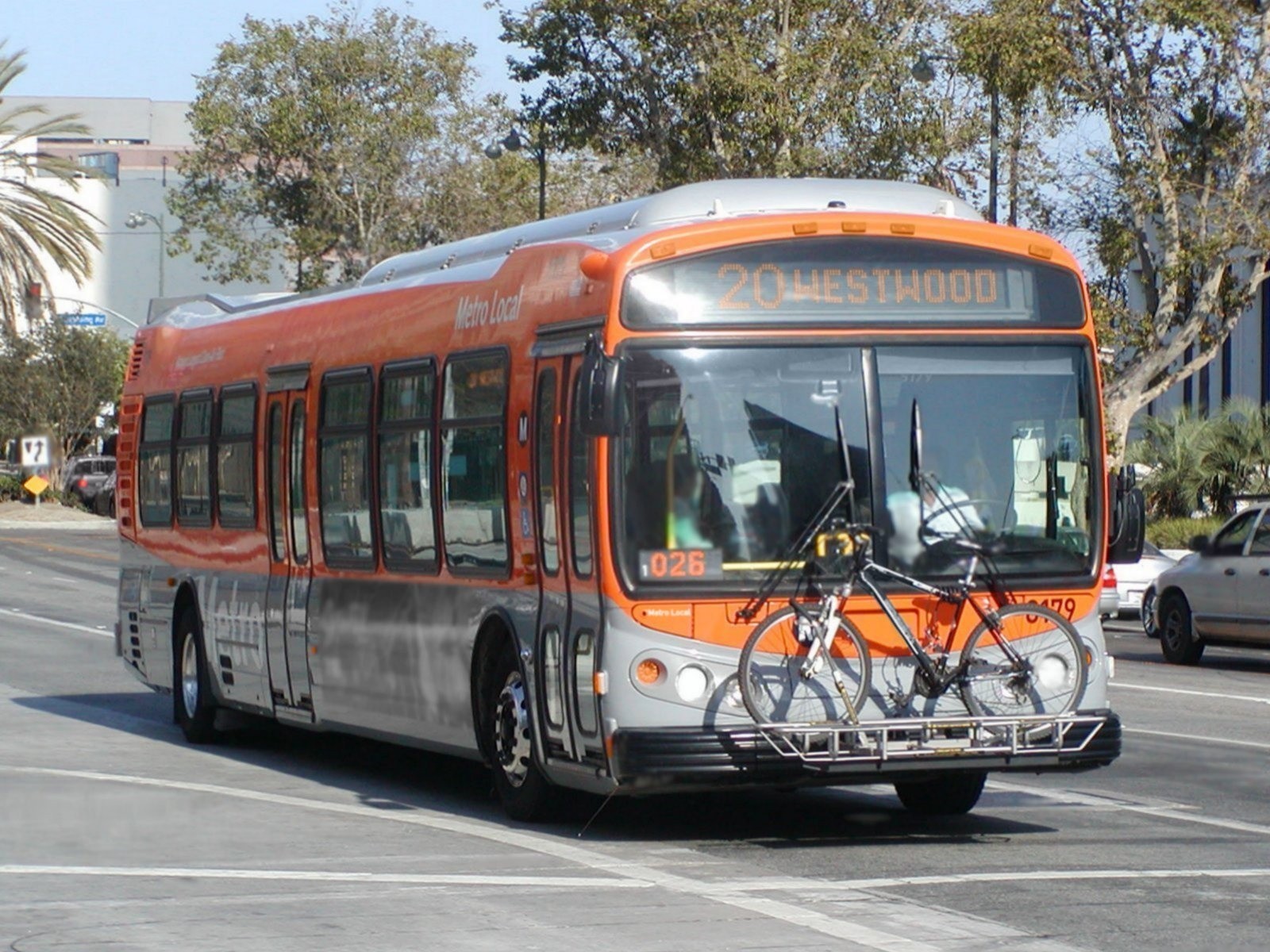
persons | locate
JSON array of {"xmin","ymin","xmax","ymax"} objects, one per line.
[{"xmin": 885, "ymin": 440, "xmax": 983, "ymax": 567}]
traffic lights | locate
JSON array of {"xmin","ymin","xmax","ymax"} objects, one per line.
[{"xmin": 26, "ymin": 282, "xmax": 42, "ymax": 319}]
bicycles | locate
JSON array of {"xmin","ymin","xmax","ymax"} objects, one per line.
[{"xmin": 737, "ymin": 518, "xmax": 1089, "ymax": 743}]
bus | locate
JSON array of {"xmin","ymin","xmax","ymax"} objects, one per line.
[{"xmin": 109, "ymin": 179, "xmax": 1123, "ymax": 820}]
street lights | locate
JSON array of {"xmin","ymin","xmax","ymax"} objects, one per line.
[
  {"xmin": 485, "ymin": 132, "xmax": 547, "ymax": 222},
  {"xmin": 127, "ymin": 211, "xmax": 165, "ymax": 295}
]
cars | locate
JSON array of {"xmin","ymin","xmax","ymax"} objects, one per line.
[
  {"xmin": 1098, "ymin": 501, "xmax": 1270, "ymax": 664},
  {"xmin": 59, "ymin": 455, "xmax": 116, "ymax": 519}
]
